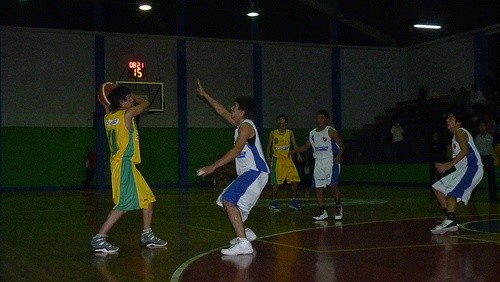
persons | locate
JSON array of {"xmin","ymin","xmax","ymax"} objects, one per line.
[
  {"xmin": 195, "ymin": 78, "xmax": 270, "ymax": 255},
  {"xmin": 289, "ymin": 109, "xmax": 345, "ymax": 221},
  {"xmin": 82, "ymin": 146, "xmax": 97, "ymax": 190},
  {"xmin": 214, "ymin": 144, "xmax": 312, "ymax": 191},
  {"xmin": 267, "ymin": 114, "xmax": 303, "ymax": 210},
  {"xmin": 428, "ymin": 110, "xmax": 500, "ymax": 234},
  {"xmin": 89, "ymin": 86, "xmax": 168, "ymax": 253}
]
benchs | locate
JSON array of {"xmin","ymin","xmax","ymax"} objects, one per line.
[{"xmin": 341, "ymin": 94, "xmax": 448, "ymax": 161}]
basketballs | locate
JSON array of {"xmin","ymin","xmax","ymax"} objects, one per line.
[{"xmin": 98, "ymin": 82, "xmax": 116, "ymax": 107}]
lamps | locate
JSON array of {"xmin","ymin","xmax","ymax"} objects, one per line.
[
  {"xmin": 138, "ymin": 0, "xmax": 152, "ymax": 11},
  {"xmin": 245, "ymin": 0, "xmax": 259, "ymax": 17}
]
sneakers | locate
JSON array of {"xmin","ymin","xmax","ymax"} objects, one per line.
[
  {"xmin": 230, "ymin": 228, "xmax": 257, "ymax": 244},
  {"xmin": 335, "ymin": 205, "xmax": 343, "ymax": 219},
  {"xmin": 289, "ymin": 199, "xmax": 301, "ymax": 210},
  {"xmin": 312, "ymin": 210, "xmax": 328, "ymax": 221},
  {"xmin": 430, "ymin": 216, "xmax": 458, "ymax": 235},
  {"xmin": 221, "ymin": 237, "xmax": 253, "ymax": 255},
  {"xmin": 88, "ymin": 236, "xmax": 119, "ymax": 252},
  {"xmin": 139, "ymin": 230, "xmax": 167, "ymax": 247},
  {"xmin": 268, "ymin": 200, "xmax": 280, "ymax": 210}
]
